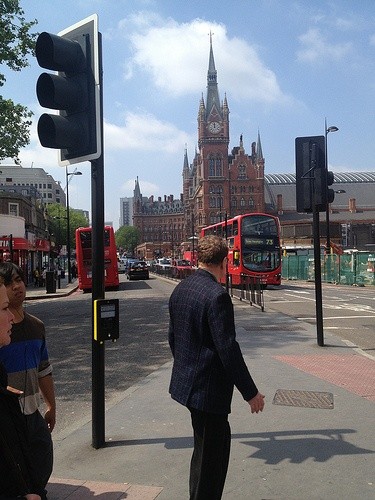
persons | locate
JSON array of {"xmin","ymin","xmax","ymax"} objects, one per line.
[
  {"xmin": 0, "ymin": 261, "xmax": 56, "ymax": 500},
  {"xmin": 34, "ymin": 267, "xmax": 39, "ymax": 288},
  {"xmin": 71, "ymin": 263, "xmax": 77, "ymax": 279},
  {"xmin": 168, "ymin": 235, "xmax": 266, "ymax": 500},
  {"xmin": 41, "ymin": 266, "xmax": 46, "ymax": 287}
]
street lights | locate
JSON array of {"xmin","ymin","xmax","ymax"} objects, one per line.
[
  {"xmin": 323, "ymin": 118, "xmax": 346, "ymax": 282},
  {"xmin": 53, "ymin": 167, "xmax": 83, "ymax": 282}
]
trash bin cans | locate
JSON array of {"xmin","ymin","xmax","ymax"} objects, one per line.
[{"xmin": 46, "ymin": 271, "xmax": 55, "ymax": 294}]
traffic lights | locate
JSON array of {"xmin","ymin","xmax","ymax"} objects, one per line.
[
  {"xmin": 33, "ymin": 13, "xmax": 105, "ymax": 166},
  {"xmin": 295, "ymin": 135, "xmax": 336, "ymax": 214}
]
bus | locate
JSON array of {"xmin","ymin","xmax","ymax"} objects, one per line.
[
  {"xmin": 76, "ymin": 225, "xmax": 120, "ymax": 292},
  {"xmin": 202, "ymin": 212, "xmax": 287, "ymax": 290}
]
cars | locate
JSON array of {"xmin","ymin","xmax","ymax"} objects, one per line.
[{"xmin": 117, "ymin": 256, "xmax": 196, "ymax": 281}]
